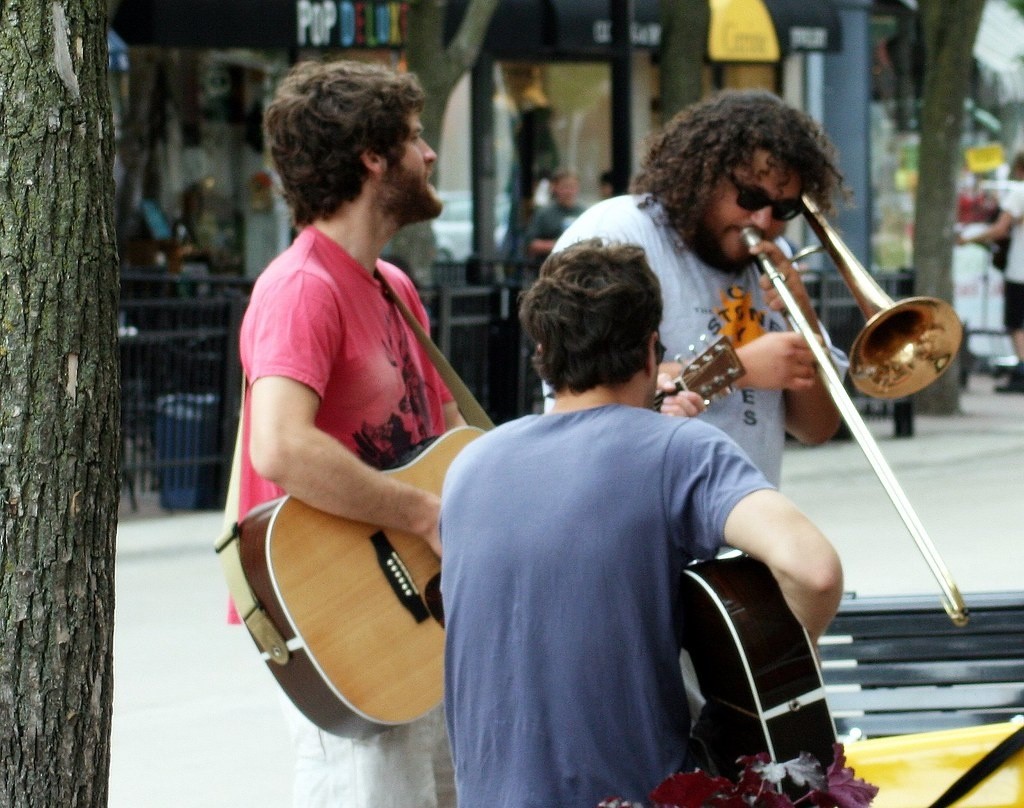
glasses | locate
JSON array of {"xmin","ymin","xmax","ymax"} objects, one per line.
[{"xmin": 723, "ymin": 167, "xmax": 803, "ymax": 222}]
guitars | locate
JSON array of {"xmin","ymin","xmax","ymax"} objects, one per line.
[
  {"xmin": 678, "ymin": 550, "xmax": 842, "ymax": 805},
  {"xmin": 229, "ymin": 329, "xmax": 750, "ymax": 746}
]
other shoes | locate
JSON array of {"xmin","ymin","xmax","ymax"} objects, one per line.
[{"xmin": 994, "ymin": 373, "xmax": 1024, "ymax": 394}]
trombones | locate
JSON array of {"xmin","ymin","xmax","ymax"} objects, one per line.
[{"xmin": 741, "ymin": 190, "xmax": 976, "ymax": 627}]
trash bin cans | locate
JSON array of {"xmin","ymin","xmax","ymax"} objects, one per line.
[{"xmin": 154, "ymin": 391, "xmax": 223, "ymax": 510}]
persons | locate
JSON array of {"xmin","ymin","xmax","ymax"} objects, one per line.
[
  {"xmin": 442, "ymin": 238, "xmax": 844, "ymax": 808},
  {"xmin": 954, "ymin": 149, "xmax": 1024, "ymax": 395},
  {"xmin": 140, "ymin": 169, "xmax": 235, "ymax": 270},
  {"xmin": 518, "ymin": 164, "xmax": 628, "ymax": 413},
  {"xmin": 795, "ymin": 249, "xmax": 824, "ymax": 319},
  {"xmin": 227, "ymin": 61, "xmax": 709, "ymax": 808},
  {"xmin": 542, "ymin": 86, "xmax": 830, "ymax": 493}
]
